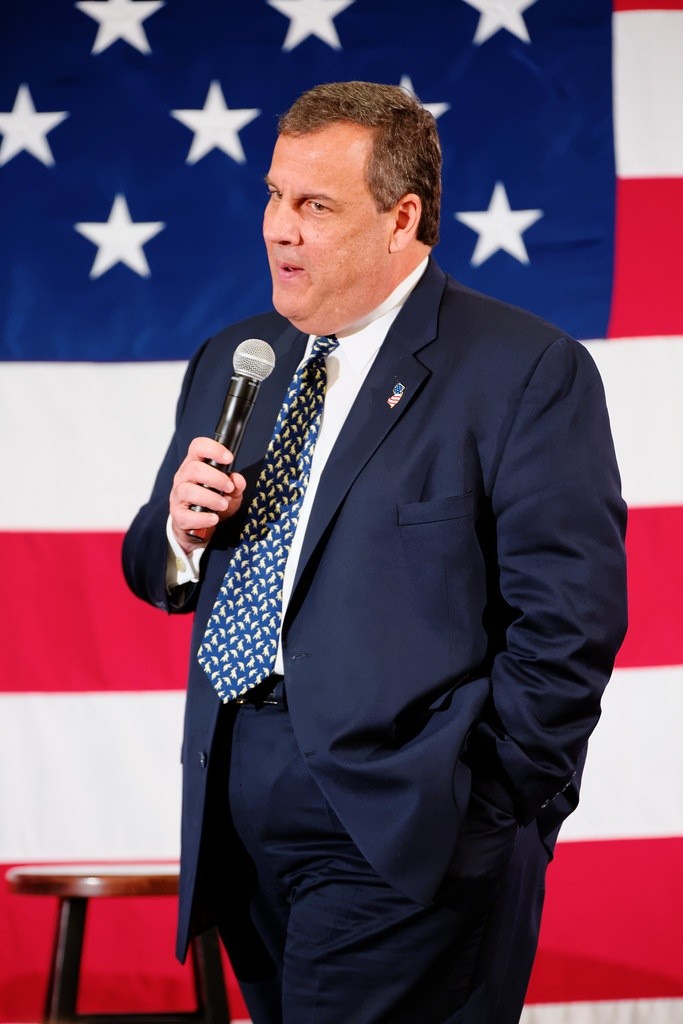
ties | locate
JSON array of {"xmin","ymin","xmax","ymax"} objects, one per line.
[{"xmin": 196, "ymin": 334, "xmax": 340, "ymax": 704}]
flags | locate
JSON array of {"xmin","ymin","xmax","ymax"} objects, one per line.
[{"xmin": 0, "ymin": 0, "xmax": 683, "ymax": 1024}]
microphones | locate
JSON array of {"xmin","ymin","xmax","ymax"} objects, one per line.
[{"xmin": 185, "ymin": 338, "xmax": 276, "ymax": 543}]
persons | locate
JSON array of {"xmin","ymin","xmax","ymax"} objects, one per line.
[{"xmin": 121, "ymin": 83, "xmax": 631, "ymax": 1024}]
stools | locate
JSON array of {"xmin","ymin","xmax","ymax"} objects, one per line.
[{"xmin": 5, "ymin": 865, "xmax": 230, "ymax": 1024}]
